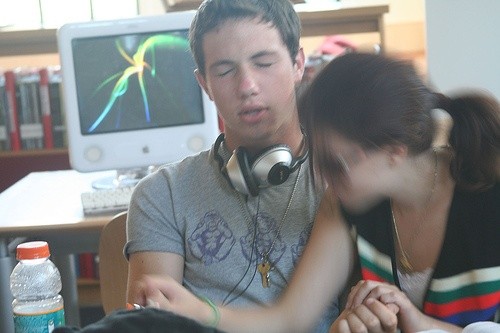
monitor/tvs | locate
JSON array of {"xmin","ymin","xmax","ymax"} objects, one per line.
[{"xmin": 55, "ymin": 10, "xmax": 219, "ymax": 189}]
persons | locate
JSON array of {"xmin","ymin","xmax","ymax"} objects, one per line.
[
  {"xmin": 132, "ymin": 51, "xmax": 500, "ymax": 333},
  {"xmin": 53, "ymin": 0, "xmax": 400, "ymax": 333}
]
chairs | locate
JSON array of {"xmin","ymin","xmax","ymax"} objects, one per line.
[{"xmin": 99, "ymin": 210, "xmax": 129, "ymax": 315}]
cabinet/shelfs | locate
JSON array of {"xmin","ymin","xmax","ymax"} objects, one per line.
[{"xmin": 0, "ymin": 5, "xmax": 392, "ymax": 195}]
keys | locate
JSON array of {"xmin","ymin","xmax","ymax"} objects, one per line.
[{"xmin": 258, "ymin": 260, "xmax": 271, "ymax": 288}]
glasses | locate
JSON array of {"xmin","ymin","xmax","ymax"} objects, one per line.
[{"xmin": 316, "ymin": 140, "xmax": 376, "ymax": 181}]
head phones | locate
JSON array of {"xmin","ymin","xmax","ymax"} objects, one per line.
[{"xmin": 213, "ymin": 122, "xmax": 311, "ymax": 199}]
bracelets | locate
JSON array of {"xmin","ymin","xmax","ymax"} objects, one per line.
[{"xmin": 200, "ymin": 295, "xmax": 219, "ymax": 327}]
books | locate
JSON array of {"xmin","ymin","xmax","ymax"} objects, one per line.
[{"xmin": 0, "ymin": 64, "xmax": 65, "ymax": 151}]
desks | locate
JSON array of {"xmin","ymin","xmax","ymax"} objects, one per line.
[{"xmin": 0, "ymin": 169, "xmax": 117, "ymax": 333}]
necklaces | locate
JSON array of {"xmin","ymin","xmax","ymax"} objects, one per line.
[{"xmin": 392, "ymin": 151, "xmax": 437, "ymax": 273}]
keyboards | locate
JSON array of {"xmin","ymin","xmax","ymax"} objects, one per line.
[{"xmin": 81, "ymin": 188, "xmax": 137, "ymax": 216}]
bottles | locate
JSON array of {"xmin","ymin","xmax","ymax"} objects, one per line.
[{"xmin": 11, "ymin": 241, "xmax": 65, "ymax": 332}]
